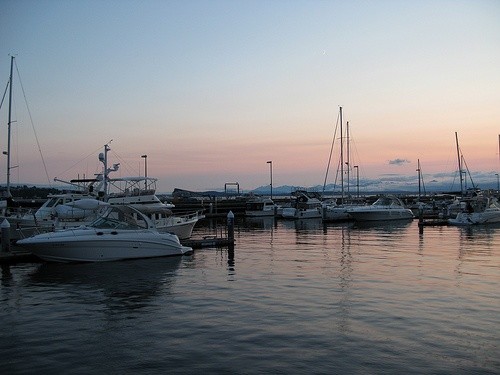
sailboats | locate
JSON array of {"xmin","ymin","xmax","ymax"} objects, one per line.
[
  {"xmin": 157, "ymin": 105, "xmax": 500, "ymax": 226},
  {"xmin": 0, "ymin": 51, "xmax": 208, "ymax": 267}
]
